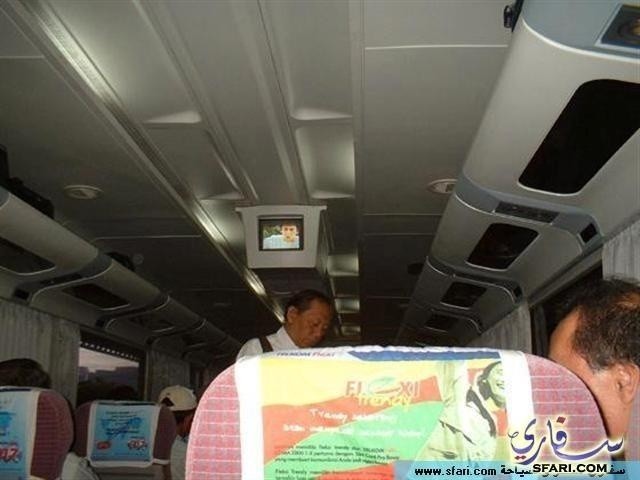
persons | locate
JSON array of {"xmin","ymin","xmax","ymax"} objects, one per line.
[
  {"xmin": 234, "ymin": 287, "xmax": 338, "ymax": 362},
  {"xmin": 0, "ymin": 358, "xmax": 101, "ymax": 480},
  {"xmin": 408, "ymin": 359, "xmax": 506, "ymax": 479},
  {"xmin": 157, "ymin": 384, "xmax": 198, "ymax": 480},
  {"xmin": 547, "ymin": 273, "xmax": 640, "ymax": 480},
  {"xmin": 263, "ymin": 221, "xmax": 300, "ymax": 250}
]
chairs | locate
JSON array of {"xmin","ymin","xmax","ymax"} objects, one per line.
[{"xmin": 0, "ymin": 345, "xmax": 613, "ymax": 479}]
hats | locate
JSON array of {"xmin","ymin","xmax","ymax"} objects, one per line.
[{"xmin": 157, "ymin": 386, "xmax": 198, "ymax": 412}]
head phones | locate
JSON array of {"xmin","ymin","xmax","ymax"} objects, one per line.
[{"xmin": 477, "ymin": 361, "xmax": 501, "ymax": 400}]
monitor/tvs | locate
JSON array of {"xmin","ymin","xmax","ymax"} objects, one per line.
[{"xmin": 259, "ymin": 219, "xmax": 304, "ymax": 251}]
